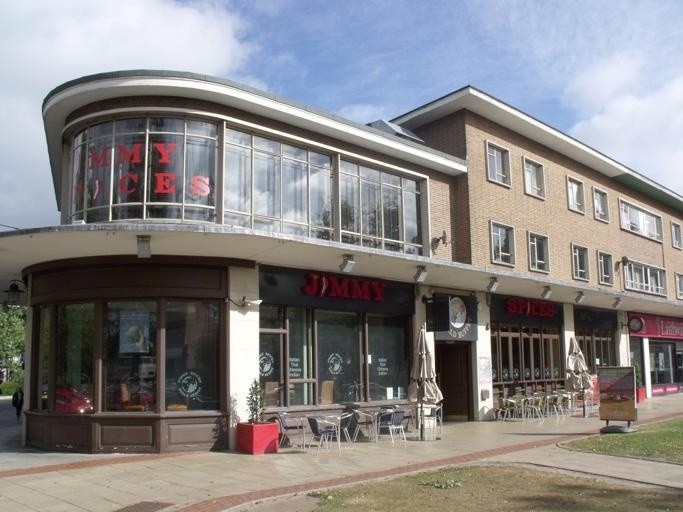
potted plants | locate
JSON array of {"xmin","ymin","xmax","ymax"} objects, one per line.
[
  {"xmin": 631, "ymin": 358, "xmax": 646, "ymax": 403},
  {"xmin": 238, "ymin": 378, "xmax": 280, "ymax": 454}
]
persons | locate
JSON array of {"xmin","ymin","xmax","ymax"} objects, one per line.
[{"xmin": 12, "ymin": 388, "xmax": 24, "ymax": 422}]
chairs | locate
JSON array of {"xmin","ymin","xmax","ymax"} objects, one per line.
[
  {"xmin": 319, "ymin": 380, "xmax": 334, "ymax": 405},
  {"xmin": 424, "ymin": 403, "xmax": 443, "ymax": 440},
  {"xmin": 167, "ymin": 386, "xmax": 189, "ymax": 410},
  {"xmin": 119, "ymin": 383, "xmax": 145, "ymax": 411},
  {"xmin": 495, "ymin": 389, "xmax": 594, "ymax": 424},
  {"xmin": 264, "ymin": 379, "xmax": 280, "ymax": 409},
  {"xmin": 278, "ymin": 404, "xmax": 408, "ymax": 456}
]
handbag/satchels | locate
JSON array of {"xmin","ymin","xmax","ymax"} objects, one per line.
[{"xmin": 12, "ymin": 400, "xmax": 17, "ymax": 406}]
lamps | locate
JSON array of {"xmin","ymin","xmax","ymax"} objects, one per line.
[
  {"xmin": 432, "ymin": 230, "xmax": 447, "ymax": 248},
  {"xmin": 136, "ymin": 235, "xmax": 151, "ymax": 258},
  {"xmin": 575, "ymin": 291, "xmax": 584, "ymax": 304},
  {"xmin": 223, "ymin": 295, "xmax": 263, "ymax": 309},
  {"xmin": 487, "ymin": 276, "xmax": 499, "ymax": 293},
  {"xmin": 339, "ymin": 254, "xmax": 356, "ymax": 273},
  {"xmin": 541, "ymin": 286, "xmax": 552, "ymax": 298},
  {"xmin": 414, "ymin": 265, "xmax": 428, "ymax": 282},
  {"xmin": 617, "ymin": 255, "xmax": 629, "ymax": 268},
  {"xmin": 3, "ymin": 279, "xmax": 25, "ymax": 307},
  {"xmin": 613, "ymin": 297, "xmax": 624, "ymax": 308}
]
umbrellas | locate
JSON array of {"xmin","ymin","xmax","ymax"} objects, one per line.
[
  {"xmin": 407, "ymin": 325, "xmax": 443, "ymax": 440},
  {"xmin": 565, "ymin": 337, "xmax": 593, "ymax": 417}
]
